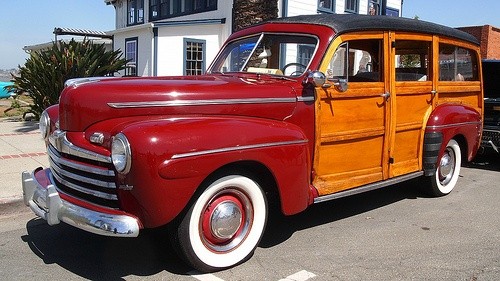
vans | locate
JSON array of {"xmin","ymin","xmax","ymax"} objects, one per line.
[{"xmin": 0, "ymin": 82, "xmax": 17, "ymax": 99}]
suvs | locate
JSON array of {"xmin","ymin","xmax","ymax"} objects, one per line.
[
  {"xmin": 22, "ymin": 15, "xmax": 487, "ymax": 271},
  {"xmin": 461, "ymin": 59, "xmax": 499, "ymax": 162}
]
